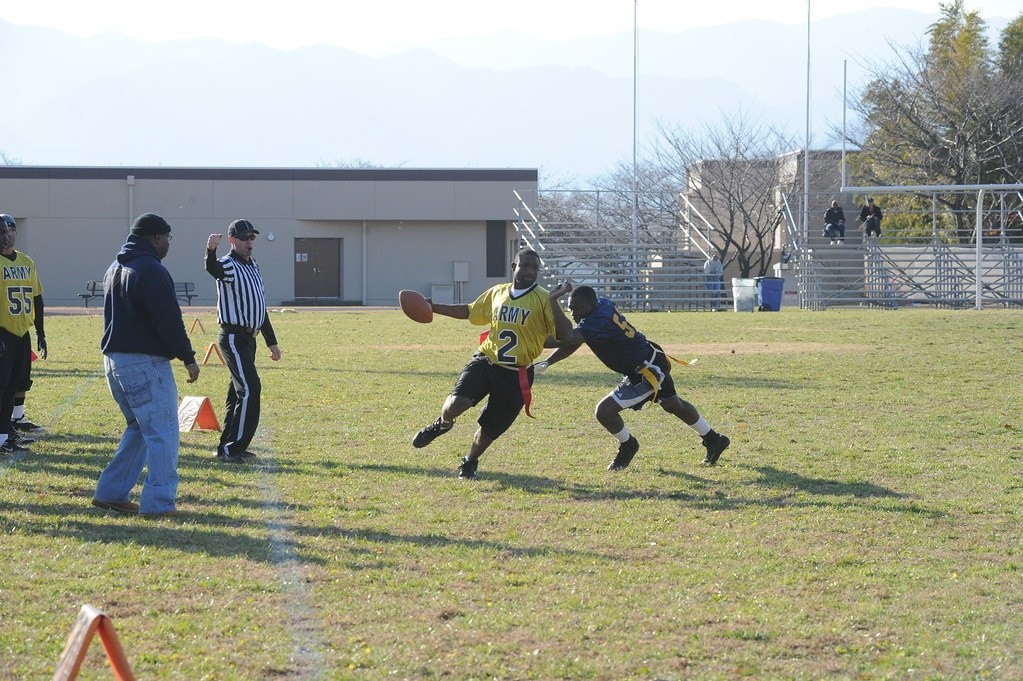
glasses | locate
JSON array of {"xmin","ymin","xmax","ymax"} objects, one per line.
[
  {"xmin": 232, "ymin": 234, "xmax": 256, "ymax": 241},
  {"xmin": 156, "ymin": 233, "xmax": 172, "ymax": 240}
]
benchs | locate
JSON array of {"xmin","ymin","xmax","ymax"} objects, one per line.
[
  {"xmin": 783, "ymin": 229, "xmax": 1023, "ymax": 311},
  {"xmin": 77, "ymin": 281, "xmax": 198, "ymax": 307},
  {"xmin": 513, "ymin": 222, "xmax": 734, "ymax": 312}
]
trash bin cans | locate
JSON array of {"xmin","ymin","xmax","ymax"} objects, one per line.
[
  {"xmin": 732, "ymin": 277, "xmax": 756, "ymax": 312},
  {"xmin": 754, "ymin": 277, "xmax": 785, "ymax": 312}
]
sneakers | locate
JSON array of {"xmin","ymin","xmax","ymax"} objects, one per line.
[
  {"xmin": 412, "ymin": 417, "xmax": 456, "ymax": 448},
  {"xmin": 699, "ymin": 429, "xmax": 730, "ymax": 468},
  {"xmin": 10, "ymin": 419, "xmax": 45, "ymax": 433},
  {"xmin": 607, "ymin": 434, "xmax": 639, "ymax": 471},
  {"xmin": 0, "ymin": 440, "xmax": 32, "ymax": 454},
  {"xmin": 8, "ymin": 434, "xmax": 35, "ymax": 445},
  {"xmin": 458, "ymin": 457, "xmax": 478, "ymax": 481}
]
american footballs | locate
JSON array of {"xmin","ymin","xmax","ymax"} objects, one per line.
[{"xmin": 399, "ymin": 290, "xmax": 434, "ymax": 324}]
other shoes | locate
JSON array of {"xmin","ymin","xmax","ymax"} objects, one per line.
[
  {"xmin": 836, "ymin": 240, "xmax": 844, "ymax": 246},
  {"xmin": 829, "ymin": 241, "xmax": 836, "ymax": 245},
  {"xmin": 91, "ymin": 499, "xmax": 140, "ymax": 513},
  {"xmin": 712, "ymin": 307, "xmax": 715, "ymax": 312},
  {"xmin": 863, "ymin": 233, "xmax": 871, "ymax": 237},
  {"xmin": 239, "ymin": 451, "xmax": 257, "ymax": 458},
  {"xmin": 876, "ymin": 233, "xmax": 885, "ymax": 237},
  {"xmin": 139, "ymin": 509, "xmax": 189, "ymax": 518},
  {"xmin": 218, "ymin": 454, "xmax": 245, "ymax": 464}
]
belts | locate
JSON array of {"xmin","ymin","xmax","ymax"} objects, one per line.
[{"xmin": 221, "ymin": 323, "xmax": 258, "ymax": 338}]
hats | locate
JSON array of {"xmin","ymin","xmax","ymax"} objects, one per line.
[
  {"xmin": 130, "ymin": 213, "xmax": 171, "ymax": 235},
  {"xmin": 228, "ymin": 218, "xmax": 260, "ymax": 237},
  {"xmin": 868, "ymin": 198, "xmax": 873, "ymax": 202},
  {"xmin": 0, "ymin": 214, "xmax": 16, "ymax": 227}
]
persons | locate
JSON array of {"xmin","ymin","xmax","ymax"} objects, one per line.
[
  {"xmin": 823, "ymin": 201, "xmax": 845, "ymax": 245},
  {"xmin": 704, "ymin": 255, "xmax": 723, "ymax": 309},
  {"xmin": 91, "ymin": 213, "xmax": 200, "ymax": 518},
  {"xmin": 0, "ymin": 214, "xmax": 47, "ymax": 454},
  {"xmin": 204, "ymin": 218, "xmax": 281, "ymax": 464},
  {"xmin": 533, "ymin": 285, "xmax": 730, "ymax": 471},
  {"xmin": 412, "ymin": 249, "xmax": 574, "ymax": 479},
  {"xmin": 860, "ymin": 198, "xmax": 883, "ymax": 237}
]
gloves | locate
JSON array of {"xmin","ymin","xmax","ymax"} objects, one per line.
[
  {"xmin": 533, "ymin": 361, "xmax": 549, "ymax": 374},
  {"xmin": 37, "ymin": 335, "xmax": 48, "ymax": 360}
]
